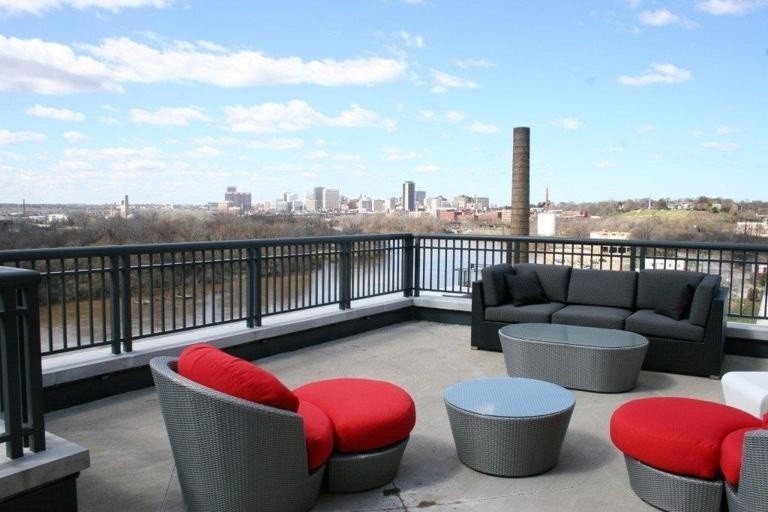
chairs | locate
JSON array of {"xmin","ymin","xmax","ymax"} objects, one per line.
[
  {"xmin": 149, "ymin": 353, "xmax": 334, "ymax": 512},
  {"xmin": 718, "ymin": 414, "xmax": 766, "ymax": 511}
]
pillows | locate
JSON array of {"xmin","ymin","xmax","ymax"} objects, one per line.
[
  {"xmin": 653, "ymin": 281, "xmax": 697, "ymax": 321},
  {"xmin": 504, "ymin": 271, "xmax": 550, "ymax": 307}
]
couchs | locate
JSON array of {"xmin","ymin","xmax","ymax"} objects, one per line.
[{"xmin": 471, "ymin": 262, "xmax": 729, "ymax": 382}]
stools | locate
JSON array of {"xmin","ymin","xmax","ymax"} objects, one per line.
[
  {"xmin": 293, "ymin": 377, "xmax": 416, "ymax": 496},
  {"xmin": 610, "ymin": 399, "xmax": 760, "ymax": 511}
]
